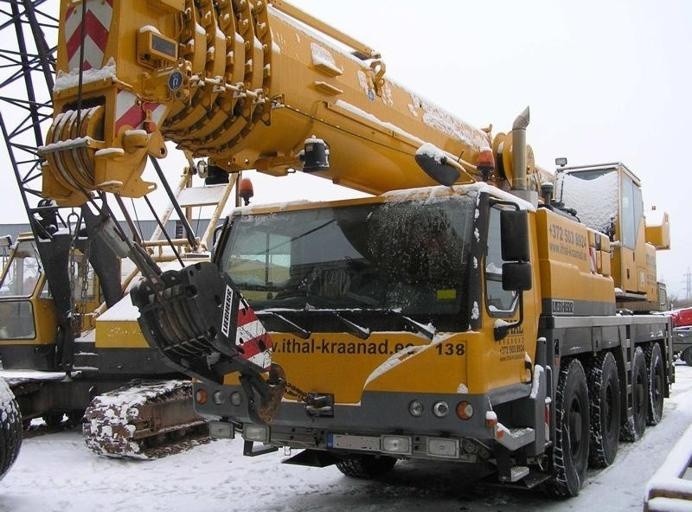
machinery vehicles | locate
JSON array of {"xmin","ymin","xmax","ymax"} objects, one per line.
[
  {"xmin": 41, "ymin": 0, "xmax": 675, "ymax": 498},
  {"xmin": 3, "ymin": 144, "xmax": 247, "ymax": 462}
]
